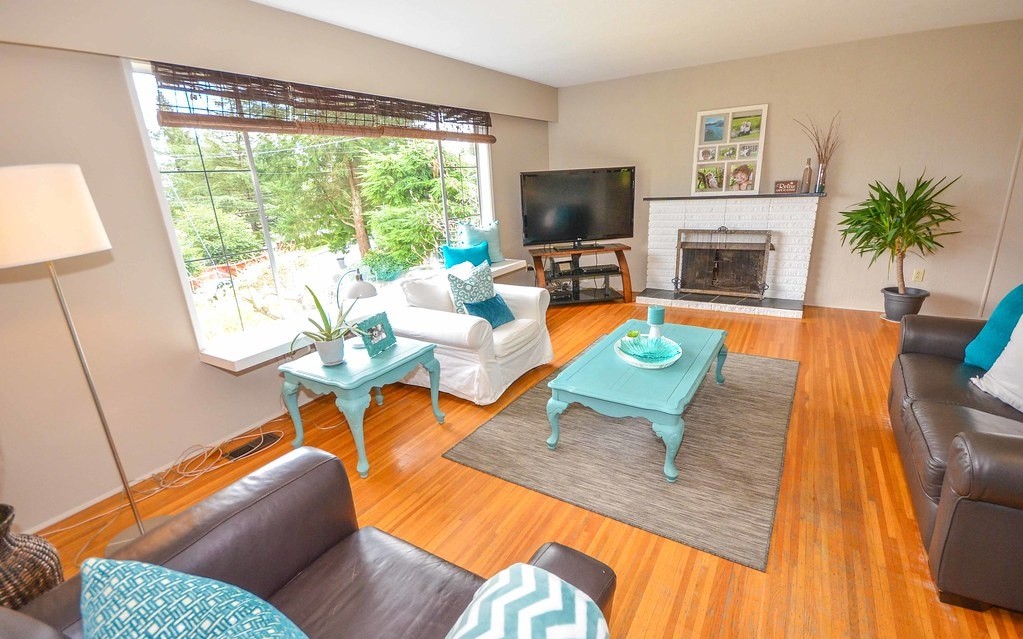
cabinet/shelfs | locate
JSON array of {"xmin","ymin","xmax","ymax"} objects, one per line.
[{"xmin": 528, "ymin": 243, "xmax": 632, "ymax": 306}]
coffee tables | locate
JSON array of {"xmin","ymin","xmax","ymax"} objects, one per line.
[{"xmin": 548, "ymin": 319, "xmax": 729, "ymax": 482}]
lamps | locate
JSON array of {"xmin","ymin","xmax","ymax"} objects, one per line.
[
  {"xmin": 337, "ymin": 267, "xmax": 378, "ymax": 348},
  {"xmin": 0, "ymin": 163, "xmax": 175, "ymax": 554}
]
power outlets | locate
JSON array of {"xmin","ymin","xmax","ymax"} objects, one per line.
[{"xmin": 912, "ymin": 268, "xmax": 925, "ymax": 282}]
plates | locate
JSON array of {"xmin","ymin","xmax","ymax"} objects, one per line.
[{"xmin": 617, "ymin": 333, "xmax": 681, "ymax": 364}]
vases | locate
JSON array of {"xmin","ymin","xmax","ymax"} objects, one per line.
[{"xmin": 815, "ymin": 164, "xmax": 829, "ymax": 192}]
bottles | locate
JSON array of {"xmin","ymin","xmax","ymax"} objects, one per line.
[{"xmin": 801, "ymin": 158, "xmax": 812, "ymax": 193}]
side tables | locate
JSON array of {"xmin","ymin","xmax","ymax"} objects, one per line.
[{"xmin": 277, "ymin": 335, "xmax": 445, "ymax": 477}]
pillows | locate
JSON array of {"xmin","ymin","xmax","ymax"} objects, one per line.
[
  {"xmin": 973, "ymin": 314, "xmax": 1023, "ymax": 413},
  {"xmin": 401, "ymin": 261, "xmax": 475, "ymax": 311},
  {"xmin": 463, "ymin": 293, "xmax": 515, "ymax": 329},
  {"xmin": 460, "ymin": 219, "xmax": 502, "ymax": 262},
  {"xmin": 966, "ymin": 281, "xmax": 1023, "ymax": 373},
  {"xmin": 448, "ymin": 259, "xmax": 496, "ymax": 313},
  {"xmin": 78, "ymin": 555, "xmax": 310, "ymax": 639},
  {"xmin": 443, "ymin": 242, "xmax": 492, "ymax": 268}
]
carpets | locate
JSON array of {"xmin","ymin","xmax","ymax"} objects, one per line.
[{"xmin": 443, "ymin": 334, "xmax": 801, "ymax": 572}]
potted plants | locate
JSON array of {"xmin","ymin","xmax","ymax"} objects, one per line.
[
  {"xmin": 838, "ymin": 165, "xmax": 962, "ymax": 322},
  {"xmin": 289, "ymin": 286, "xmax": 373, "ymax": 365}
]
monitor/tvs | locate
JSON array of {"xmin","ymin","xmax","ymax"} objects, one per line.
[{"xmin": 520, "ymin": 166, "xmax": 635, "ymax": 252}]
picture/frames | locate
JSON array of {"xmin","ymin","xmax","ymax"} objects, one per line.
[
  {"xmin": 691, "ymin": 104, "xmax": 768, "ymax": 196},
  {"xmin": 358, "ymin": 311, "xmax": 396, "ymax": 357}
]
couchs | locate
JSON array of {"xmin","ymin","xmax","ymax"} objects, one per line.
[
  {"xmin": 887, "ymin": 314, "xmax": 1023, "ymax": 616},
  {"xmin": 0, "ymin": 446, "xmax": 616, "ymax": 639},
  {"xmin": 377, "ymin": 279, "xmax": 553, "ymax": 407}
]
